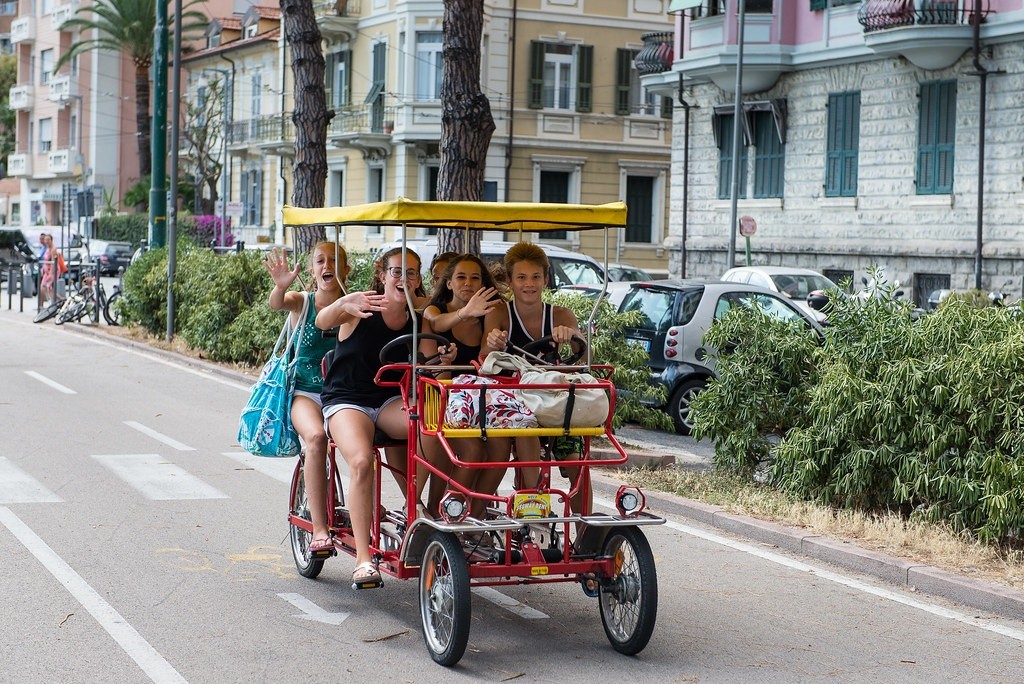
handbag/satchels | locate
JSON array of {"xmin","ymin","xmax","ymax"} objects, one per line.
[
  {"xmin": 479, "ymin": 352, "xmax": 609, "ymax": 428},
  {"xmin": 57, "ymin": 253, "xmax": 68, "ymax": 273},
  {"xmin": 444, "ymin": 373, "xmax": 539, "ymax": 430},
  {"xmin": 237, "ymin": 290, "xmax": 310, "ymax": 458}
]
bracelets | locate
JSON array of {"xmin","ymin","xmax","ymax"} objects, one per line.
[{"xmin": 456, "ymin": 309, "xmax": 463, "ymax": 321}]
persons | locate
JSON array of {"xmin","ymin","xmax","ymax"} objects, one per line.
[
  {"xmin": 423, "ymin": 243, "xmax": 600, "ymax": 597},
  {"xmin": 33, "ymin": 233, "xmax": 65, "ymax": 311},
  {"xmin": 263, "ymin": 242, "xmax": 386, "ymax": 552},
  {"xmin": 779, "ymin": 277, "xmax": 797, "ymax": 298},
  {"xmin": 423, "ymin": 254, "xmax": 509, "ymax": 544},
  {"xmin": 315, "ymin": 246, "xmax": 457, "ymax": 584}
]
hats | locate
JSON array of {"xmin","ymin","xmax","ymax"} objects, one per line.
[{"xmin": 775, "ymin": 275, "xmax": 795, "ymax": 291}]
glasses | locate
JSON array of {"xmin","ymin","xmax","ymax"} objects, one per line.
[
  {"xmin": 788, "ymin": 290, "xmax": 800, "ymax": 296},
  {"xmin": 382, "ymin": 267, "xmax": 419, "ymax": 280}
]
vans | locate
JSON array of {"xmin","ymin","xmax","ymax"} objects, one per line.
[{"xmin": 0, "ymin": 225, "xmax": 94, "ymax": 286}]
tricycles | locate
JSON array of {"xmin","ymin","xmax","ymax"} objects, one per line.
[{"xmin": 278, "ymin": 195, "xmax": 668, "ymax": 669}]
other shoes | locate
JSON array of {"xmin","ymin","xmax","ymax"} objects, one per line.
[
  {"xmin": 455, "ymin": 534, "xmax": 468, "ymax": 547},
  {"xmin": 464, "ymin": 532, "xmax": 497, "ymax": 546}
]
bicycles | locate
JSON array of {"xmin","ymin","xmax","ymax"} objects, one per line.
[{"xmin": 32, "ymin": 280, "xmax": 123, "ymax": 326}]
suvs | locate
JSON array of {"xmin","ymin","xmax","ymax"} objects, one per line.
[
  {"xmin": 82, "ymin": 238, "xmax": 131, "ymax": 276},
  {"xmin": 372, "ymin": 236, "xmax": 617, "ymax": 294}
]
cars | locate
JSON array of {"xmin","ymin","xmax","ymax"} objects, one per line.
[
  {"xmin": 597, "ymin": 260, "xmax": 653, "ymax": 283},
  {"xmin": 227, "ymin": 242, "xmax": 295, "ymax": 256},
  {"xmin": 718, "ymin": 266, "xmax": 850, "ymax": 332},
  {"xmin": 552, "ymin": 279, "xmax": 640, "ymax": 320},
  {"xmin": 611, "ymin": 279, "xmax": 825, "ymax": 437}
]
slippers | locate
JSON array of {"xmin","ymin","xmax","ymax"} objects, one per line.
[
  {"xmin": 310, "ymin": 538, "xmax": 335, "ymax": 551},
  {"xmin": 577, "ymin": 568, "xmax": 599, "ymax": 598},
  {"xmin": 353, "ymin": 563, "xmax": 381, "ymax": 584},
  {"xmin": 402, "ymin": 503, "xmax": 433, "ymax": 523}
]
